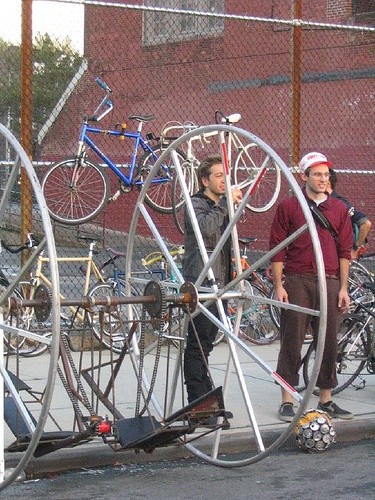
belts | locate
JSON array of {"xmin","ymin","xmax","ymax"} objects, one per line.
[{"xmin": 325, "ymin": 274, "xmax": 338, "ymax": 279}]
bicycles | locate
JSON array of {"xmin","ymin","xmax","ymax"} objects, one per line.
[
  {"xmin": 137, "ymin": 112, "xmax": 278, "ymax": 213},
  {"xmin": 40, "ymin": 77, "xmax": 192, "ymax": 227},
  {"xmin": 1, "ymin": 235, "xmax": 374, "ymax": 400}
]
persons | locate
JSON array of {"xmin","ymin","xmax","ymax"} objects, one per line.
[
  {"xmin": 324, "ymin": 167, "xmax": 372, "ymax": 261},
  {"xmin": 268, "ymin": 152, "xmax": 355, "ymax": 422},
  {"xmin": 182, "ymin": 155, "xmax": 243, "ymax": 431}
]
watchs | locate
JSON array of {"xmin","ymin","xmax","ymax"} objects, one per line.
[{"xmin": 353, "ymin": 240, "xmax": 360, "ymax": 251}]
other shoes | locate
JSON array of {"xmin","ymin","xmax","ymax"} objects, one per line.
[
  {"xmin": 191, "ymin": 410, "xmax": 234, "ymax": 429},
  {"xmin": 317, "ymin": 401, "xmax": 352, "ymax": 419},
  {"xmin": 278, "ymin": 403, "xmax": 296, "ymax": 421}
]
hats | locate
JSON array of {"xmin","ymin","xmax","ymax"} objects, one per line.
[{"xmin": 299, "ymin": 152, "xmax": 332, "ymax": 173}]
